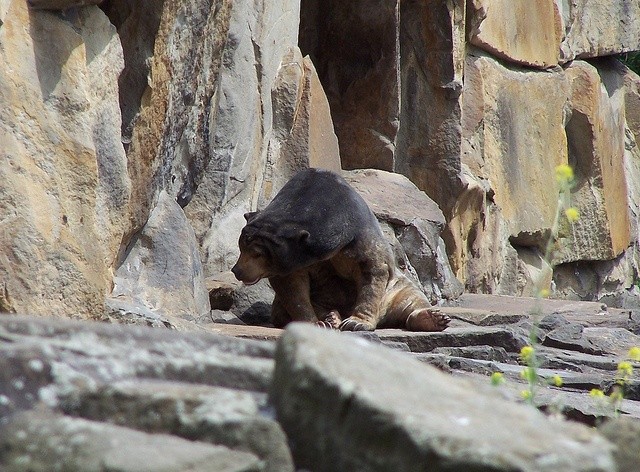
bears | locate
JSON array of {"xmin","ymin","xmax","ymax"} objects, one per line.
[{"xmin": 231, "ymin": 167, "xmax": 451, "ymax": 332}]
